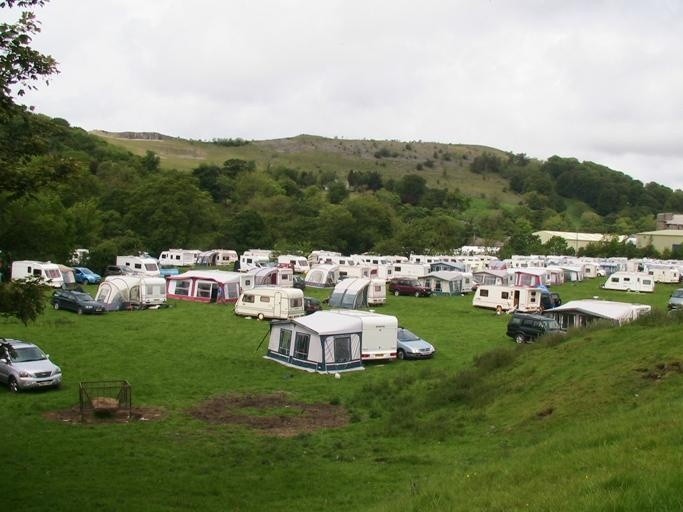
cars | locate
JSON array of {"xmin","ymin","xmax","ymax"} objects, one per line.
[
  {"xmin": 398, "ymin": 326, "xmax": 436, "ymax": 359},
  {"xmin": 0, "ymin": 337, "xmax": 62, "ymax": 392}
]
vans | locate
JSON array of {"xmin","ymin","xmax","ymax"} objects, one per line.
[{"xmin": 326, "ymin": 309, "xmax": 398, "ymax": 361}]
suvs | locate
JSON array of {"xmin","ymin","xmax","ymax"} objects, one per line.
[{"xmin": 506, "ymin": 313, "xmax": 566, "ymax": 344}]
